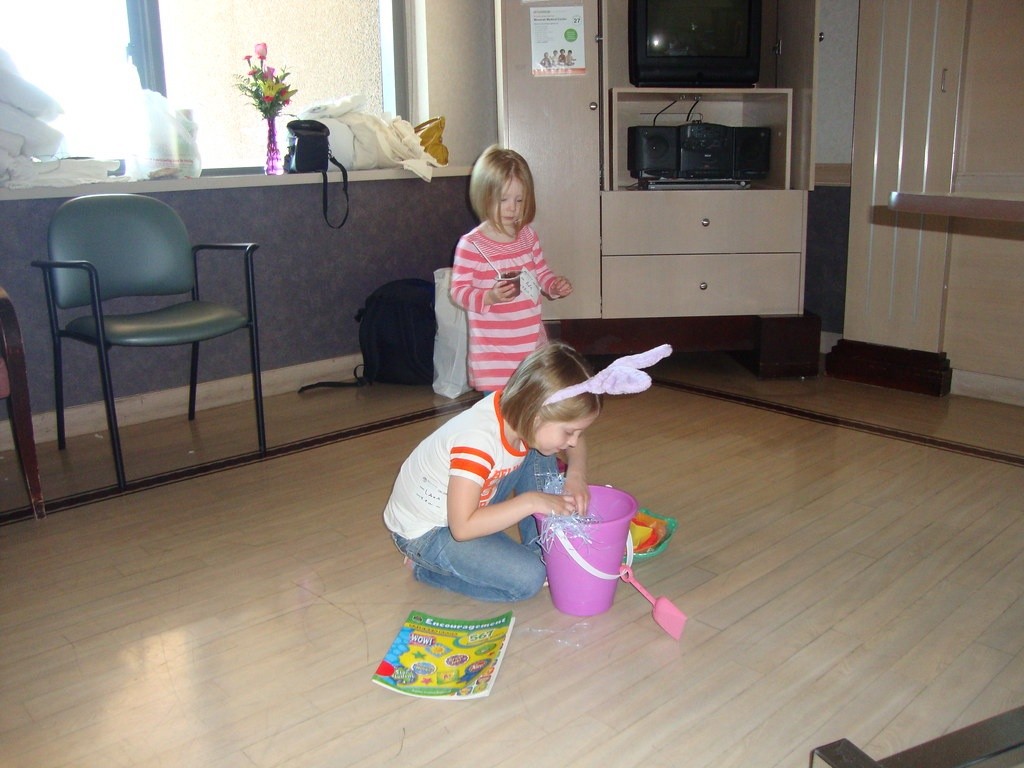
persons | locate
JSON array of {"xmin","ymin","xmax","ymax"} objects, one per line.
[
  {"xmin": 540, "ymin": 49, "xmax": 576, "ymax": 68},
  {"xmin": 383, "ymin": 344, "xmax": 603, "ymax": 602},
  {"xmin": 449, "ymin": 144, "xmax": 574, "ymax": 398}
]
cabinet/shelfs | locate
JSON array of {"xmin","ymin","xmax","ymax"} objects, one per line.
[{"xmin": 500, "ymin": 1, "xmax": 819, "ymax": 320}]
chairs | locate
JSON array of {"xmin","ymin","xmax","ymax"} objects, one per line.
[
  {"xmin": 0, "ymin": 287, "xmax": 46, "ymax": 521},
  {"xmin": 30, "ymin": 193, "xmax": 267, "ymax": 488}
]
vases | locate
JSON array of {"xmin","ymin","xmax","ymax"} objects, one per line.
[{"xmin": 264, "ymin": 117, "xmax": 284, "ymax": 175}]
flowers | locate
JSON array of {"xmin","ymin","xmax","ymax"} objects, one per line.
[{"xmin": 223, "ymin": 41, "xmax": 298, "ymax": 120}]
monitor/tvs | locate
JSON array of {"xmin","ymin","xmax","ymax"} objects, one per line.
[{"xmin": 628, "ymin": 0, "xmax": 762, "ymax": 88}]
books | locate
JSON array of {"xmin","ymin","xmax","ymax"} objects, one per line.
[{"xmin": 372, "ymin": 610, "xmax": 517, "ymax": 700}]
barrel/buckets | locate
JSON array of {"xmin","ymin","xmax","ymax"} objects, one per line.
[{"xmin": 534, "ymin": 485, "xmax": 637, "ymax": 616}]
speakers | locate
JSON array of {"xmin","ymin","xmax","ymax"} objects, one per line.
[
  {"xmin": 628, "ymin": 125, "xmax": 680, "ymax": 178},
  {"xmin": 734, "ymin": 126, "xmax": 771, "ymax": 179}
]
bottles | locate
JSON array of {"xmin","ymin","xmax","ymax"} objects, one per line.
[{"xmin": 177, "ymin": 109, "xmax": 199, "ymax": 177}]
